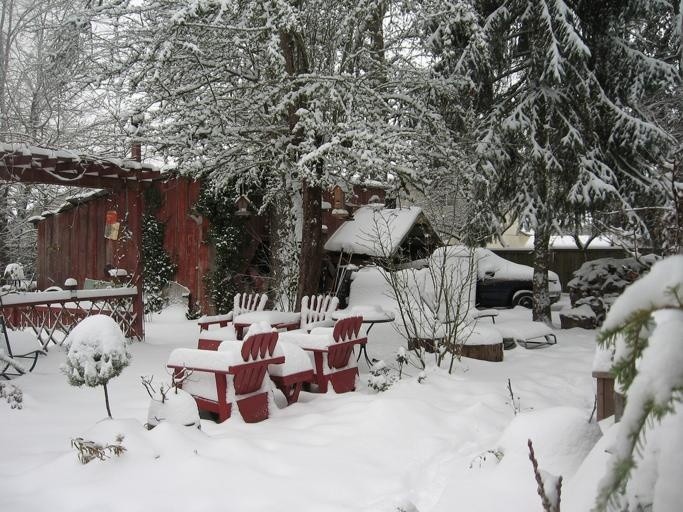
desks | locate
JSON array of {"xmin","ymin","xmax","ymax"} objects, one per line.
[{"xmin": 332, "ymin": 309, "xmax": 395, "ymax": 365}]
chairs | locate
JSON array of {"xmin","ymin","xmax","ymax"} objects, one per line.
[{"xmin": 166, "ymin": 292, "xmax": 368, "ymax": 424}]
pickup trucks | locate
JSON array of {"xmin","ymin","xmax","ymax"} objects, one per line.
[{"xmin": 384, "ymin": 247, "xmax": 561, "ymax": 310}]
